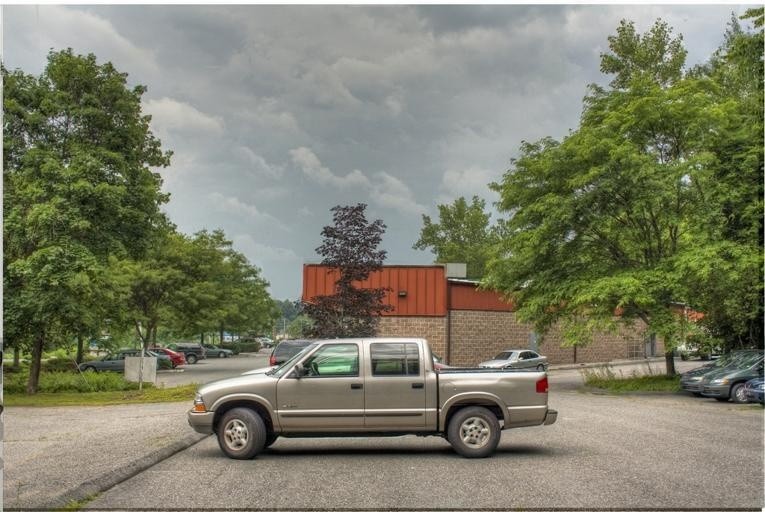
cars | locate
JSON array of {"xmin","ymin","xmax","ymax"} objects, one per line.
[
  {"xmin": 203, "ymin": 343, "xmax": 233, "ymax": 357},
  {"xmin": 255, "ymin": 337, "xmax": 274, "ymax": 348},
  {"xmin": 271, "ymin": 341, "xmax": 439, "ymax": 365},
  {"xmin": 479, "ymin": 350, "xmax": 549, "ymax": 372},
  {"xmin": 164, "ymin": 343, "xmax": 207, "ymax": 364},
  {"xmin": 680, "ymin": 348, "xmax": 765, "ymax": 405},
  {"xmin": 678, "ymin": 334, "xmax": 725, "ymax": 360},
  {"xmin": 79, "ymin": 350, "xmax": 186, "ymax": 373}
]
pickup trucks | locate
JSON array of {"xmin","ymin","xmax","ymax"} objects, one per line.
[{"xmin": 187, "ymin": 338, "xmax": 558, "ymax": 460}]
